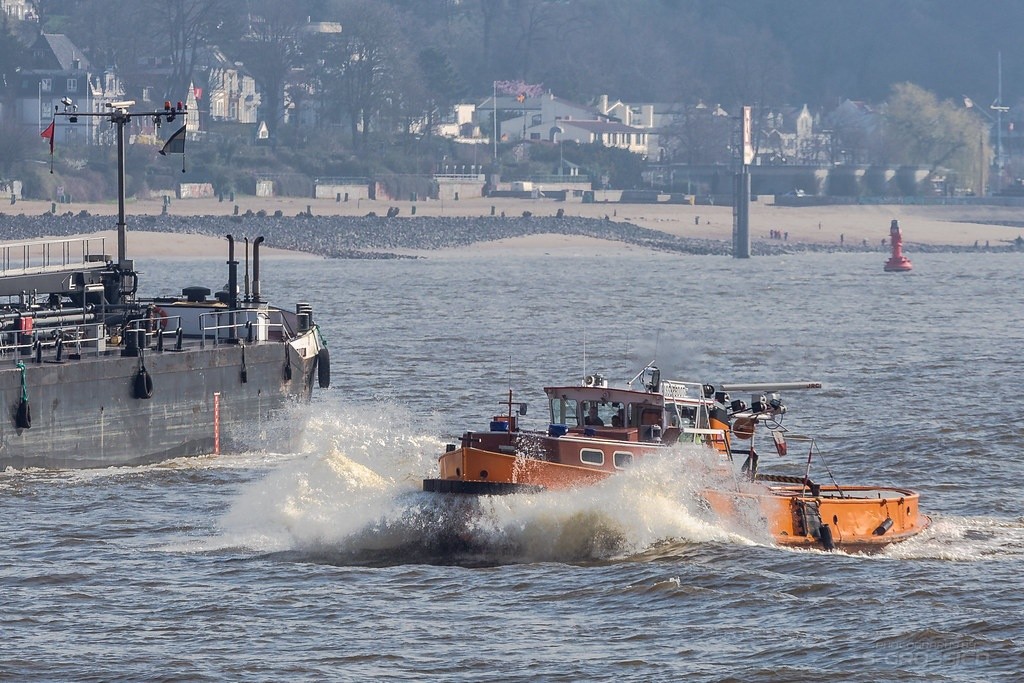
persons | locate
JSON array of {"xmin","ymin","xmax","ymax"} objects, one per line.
[
  {"xmin": 611, "ymin": 415, "xmax": 620, "ymax": 426},
  {"xmin": 584, "ymin": 406, "xmax": 604, "ymax": 427}
]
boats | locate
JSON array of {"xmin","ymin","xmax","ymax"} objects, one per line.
[
  {"xmin": 415, "ymin": 362, "xmax": 939, "ymax": 549},
  {"xmin": 0, "ymin": 93, "xmax": 333, "ymax": 461}
]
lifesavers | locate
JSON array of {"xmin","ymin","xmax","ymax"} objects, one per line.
[{"xmin": 146, "ymin": 307, "xmax": 168, "ymax": 333}]
